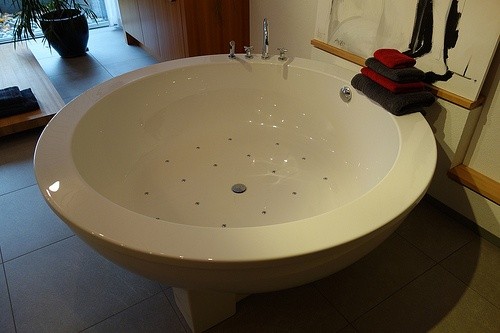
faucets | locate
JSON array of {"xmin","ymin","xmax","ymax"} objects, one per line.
[{"xmin": 260, "ymin": 14, "xmax": 272, "ymax": 58}]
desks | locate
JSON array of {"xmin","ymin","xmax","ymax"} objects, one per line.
[{"xmin": 118, "ymin": 0, "xmax": 250, "ymax": 63}]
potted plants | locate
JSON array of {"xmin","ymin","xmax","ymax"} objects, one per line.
[{"xmin": 2, "ymin": 0, "xmax": 99, "ymax": 58}]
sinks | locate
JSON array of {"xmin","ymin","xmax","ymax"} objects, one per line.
[{"xmin": 31, "ymin": 53, "xmax": 439, "ymax": 292}]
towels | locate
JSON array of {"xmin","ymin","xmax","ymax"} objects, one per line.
[{"xmin": 350, "ymin": 42, "xmax": 439, "ymax": 119}]
